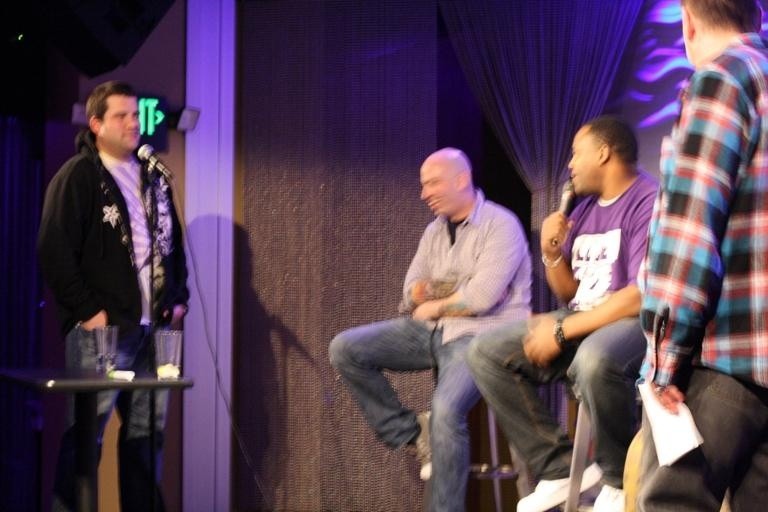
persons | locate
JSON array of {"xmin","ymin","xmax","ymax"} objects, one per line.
[
  {"xmin": 466, "ymin": 114, "xmax": 660, "ymax": 512},
  {"xmin": 328, "ymin": 147, "xmax": 533, "ymax": 511},
  {"xmin": 636, "ymin": 1, "xmax": 768, "ymax": 510},
  {"xmin": 34, "ymin": 82, "xmax": 191, "ymax": 512}
]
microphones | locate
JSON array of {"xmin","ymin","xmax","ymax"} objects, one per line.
[
  {"xmin": 137, "ymin": 144, "xmax": 170, "ymax": 177},
  {"xmin": 553, "ymin": 181, "xmax": 575, "ymax": 246}
]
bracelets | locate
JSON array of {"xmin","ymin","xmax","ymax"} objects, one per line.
[
  {"xmin": 542, "ymin": 253, "xmax": 563, "ymax": 267},
  {"xmin": 554, "ymin": 320, "xmax": 567, "ymax": 347}
]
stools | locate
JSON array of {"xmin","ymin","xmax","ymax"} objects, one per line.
[
  {"xmin": 467, "ymin": 385, "xmax": 520, "ymax": 512},
  {"xmin": 561, "ymin": 390, "xmax": 591, "ymax": 512}
]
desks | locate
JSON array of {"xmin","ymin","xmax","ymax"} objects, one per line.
[{"xmin": 0, "ymin": 368, "xmax": 194, "ymax": 512}]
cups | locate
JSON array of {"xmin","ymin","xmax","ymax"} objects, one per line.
[
  {"xmin": 154, "ymin": 331, "xmax": 183, "ymax": 381},
  {"xmin": 94, "ymin": 325, "xmax": 117, "ymax": 373}
]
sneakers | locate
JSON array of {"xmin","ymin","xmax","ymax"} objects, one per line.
[
  {"xmin": 514, "ymin": 461, "xmax": 626, "ymax": 512},
  {"xmin": 412, "ymin": 410, "xmax": 431, "ymax": 481}
]
